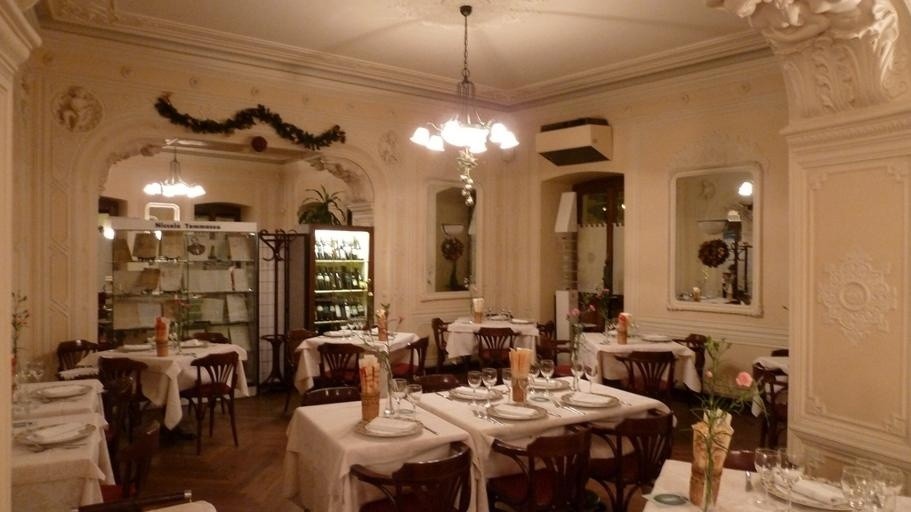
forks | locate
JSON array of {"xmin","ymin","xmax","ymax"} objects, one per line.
[{"xmin": 552, "ymin": 398, "xmax": 585, "ymax": 417}]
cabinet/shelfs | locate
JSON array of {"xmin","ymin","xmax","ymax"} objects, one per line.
[
  {"xmin": 187, "ymin": 226, "xmax": 261, "ymax": 395},
  {"xmin": 111, "ymin": 220, "xmax": 189, "ymax": 346}
]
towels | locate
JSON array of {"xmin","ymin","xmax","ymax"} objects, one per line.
[{"xmin": 776, "ymin": 469, "xmax": 849, "ymax": 505}]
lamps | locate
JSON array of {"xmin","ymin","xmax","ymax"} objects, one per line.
[
  {"xmin": 410, "ymin": 4, "xmax": 523, "ymax": 210},
  {"xmin": 141, "ymin": 137, "xmax": 207, "ymax": 199},
  {"xmin": 98, "ymin": 222, "xmax": 117, "ymax": 240}
]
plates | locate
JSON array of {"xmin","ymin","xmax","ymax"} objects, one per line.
[
  {"xmin": 487, "ymin": 402, "xmax": 547, "ymax": 420},
  {"xmin": 527, "ymin": 377, "xmax": 571, "ymax": 389},
  {"xmin": 761, "ymin": 473, "xmax": 851, "ymax": 512},
  {"xmin": 121, "ymin": 345, "xmax": 153, "ymax": 352},
  {"xmin": 642, "ymin": 335, "xmax": 673, "ymax": 343},
  {"xmin": 33, "ymin": 383, "xmax": 93, "ymax": 400},
  {"xmin": 512, "ymin": 319, "xmax": 535, "ymax": 324},
  {"xmin": 561, "ymin": 391, "xmax": 619, "ymax": 407},
  {"xmin": 14, "ymin": 423, "xmax": 95, "ymax": 446},
  {"xmin": 325, "ymin": 329, "xmax": 353, "ymax": 337},
  {"xmin": 353, "ymin": 416, "xmax": 424, "ymax": 437},
  {"xmin": 182, "ymin": 340, "xmax": 210, "ymax": 348},
  {"xmin": 365, "ymin": 331, "xmax": 379, "ymax": 336},
  {"xmin": 449, "ymin": 387, "xmax": 505, "ymax": 402}
]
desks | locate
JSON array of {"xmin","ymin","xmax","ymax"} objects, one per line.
[
  {"xmin": 293, "ymin": 397, "xmax": 471, "ymax": 512},
  {"xmin": 447, "ymin": 317, "xmax": 538, "ymax": 368},
  {"xmin": 753, "ymin": 357, "xmax": 790, "ymax": 376},
  {"xmin": 405, "ymin": 376, "xmax": 662, "ymax": 512},
  {"xmin": 575, "ymin": 331, "xmax": 695, "ymax": 381},
  {"xmin": 12, "ymin": 378, "xmax": 99, "ymax": 512}
]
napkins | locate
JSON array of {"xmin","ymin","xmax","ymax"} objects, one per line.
[
  {"xmin": 455, "ymin": 385, "xmax": 496, "ymax": 398},
  {"xmin": 522, "ymin": 378, "xmax": 565, "ymax": 389},
  {"xmin": 34, "ymin": 421, "xmax": 86, "ymax": 437}
]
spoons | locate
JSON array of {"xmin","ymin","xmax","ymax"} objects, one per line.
[{"xmin": 471, "ymin": 409, "xmax": 503, "ymax": 428}]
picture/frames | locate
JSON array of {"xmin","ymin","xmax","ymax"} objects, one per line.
[
  {"xmin": 667, "ymin": 159, "xmax": 764, "ymax": 318},
  {"xmin": 415, "ymin": 177, "xmax": 485, "ymax": 303}
]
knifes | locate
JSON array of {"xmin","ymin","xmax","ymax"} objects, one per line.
[
  {"xmin": 421, "ymin": 423, "xmax": 439, "ymax": 436},
  {"xmin": 744, "ymin": 470, "xmax": 754, "ymax": 492},
  {"xmin": 435, "ymin": 390, "xmax": 453, "ymax": 400}
]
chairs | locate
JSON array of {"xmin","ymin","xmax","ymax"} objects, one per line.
[
  {"xmin": 475, "ymin": 326, "xmax": 517, "ymax": 368},
  {"xmin": 613, "ymin": 351, "xmax": 676, "ymax": 402},
  {"xmin": 749, "ymin": 368, "xmax": 789, "ymax": 450},
  {"xmin": 55, "ymin": 332, "xmax": 238, "ymax": 461},
  {"xmin": 772, "ymin": 349, "xmax": 788, "ymax": 357},
  {"xmin": 351, "ymin": 409, "xmax": 675, "ymax": 511},
  {"xmin": 284, "ymin": 318, "xmax": 461, "ymax": 401},
  {"xmin": 576, "ymin": 312, "xmax": 607, "ymax": 333},
  {"xmin": 537, "ymin": 326, "xmax": 575, "ymax": 366},
  {"xmin": 674, "ymin": 332, "xmax": 708, "ymax": 379}
]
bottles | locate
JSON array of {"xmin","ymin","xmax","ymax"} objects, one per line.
[{"xmin": 315, "ymin": 237, "xmax": 365, "ymax": 319}]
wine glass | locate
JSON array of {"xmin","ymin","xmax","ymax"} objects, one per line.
[
  {"xmin": 391, "ymin": 378, "xmax": 407, "ymax": 418},
  {"xmin": 487, "ymin": 305, "xmax": 512, "ymax": 323},
  {"xmin": 528, "ymin": 359, "xmax": 555, "ymax": 396},
  {"xmin": 467, "ymin": 368, "xmax": 513, "ymax": 395},
  {"xmin": 570, "ymin": 359, "xmax": 585, "ymax": 391},
  {"xmin": 608, "ymin": 318, "xmax": 618, "ymax": 340},
  {"xmin": 519, "ymin": 374, "xmax": 536, "ymax": 404},
  {"xmin": 629, "ymin": 320, "xmax": 641, "ymax": 340},
  {"xmin": 338, "ymin": 317, "xmax": 367, "ymax": 336},
  {"xmin": 754, "ymin": 447, "xmax": 782, "ymax": 512},
  {"xmin": 778, "ymin": 447, "xmax": 806, "ymax": 512},
  {"xmin": 406, "ymin": 383, "xmax": 423, "ymax": 420},
  {"xmin": 585, "ymin": 361, "xmax": 601, "ymax": 393}
]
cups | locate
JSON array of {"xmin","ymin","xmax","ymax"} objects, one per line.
[
  {"xmin": 475, "ymin": 312, "xmax": 483, "ymax": 323},
  {"xmin": 617, "ymin": 330, "xmax": 627, "ymax": 344},
  {"xmin": 156, "ymin": 338, "xmax": 169, "ymax": 357},
  {"xmin": 512, "ymin": 376, "xmax": 528, "ymax": 401},
  {"xmin": 842, "ymin": 458, "xmax": 905, "ymax": 512},
  {"xmin": 378, "ymin": 327, "xmax": 388, "ymax": 341},
  {"xmin": 689, "ymin": 461, "xmax": 724, "ymax": 508},
  {"xmin": 15, "ymin": 358, "xmax": 45, "ymax": 421},
  {"xmin": 360, "ymin": 392, "xmax": 380, "ymax": 421}
]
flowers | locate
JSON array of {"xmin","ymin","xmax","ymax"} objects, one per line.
[
  {"xmin": 703, "ymin": 368, "xmax": 753, "ymax": 392},
  {"xmin": 11, "ymin": 286, "xmax": 31, "ymax": 359}
]
